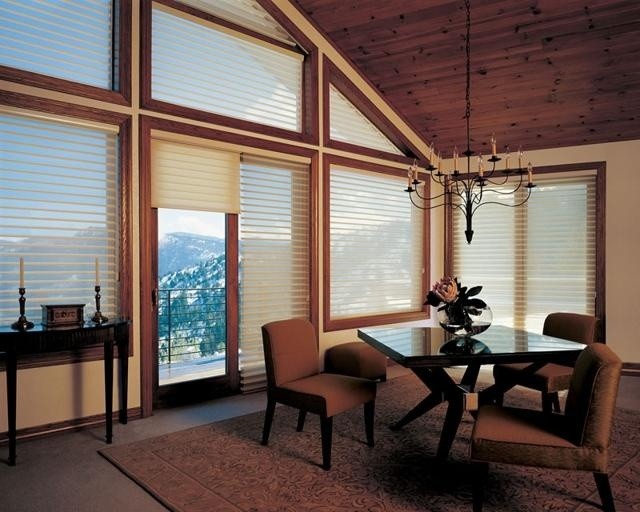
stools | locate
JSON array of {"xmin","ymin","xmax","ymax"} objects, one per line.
[{"xmin": 325, "ymin": 343, "xmax": 387, "ymax": 383}]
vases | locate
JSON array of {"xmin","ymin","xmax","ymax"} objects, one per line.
[{"xmin": 438, "ymin": 303, "xmax": 492, "ymax": 337}]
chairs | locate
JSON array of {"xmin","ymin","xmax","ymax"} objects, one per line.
[
  {"xmin": 469, "ymin": 341, "xmax": 622, "ymax": 512},
  {"xmin": 261, "ymin": 318, "xmax": 375, "ymax": 469},
  {"xmin": 493, "ymin": 312, "xmax": 602, "ymax": 412}
]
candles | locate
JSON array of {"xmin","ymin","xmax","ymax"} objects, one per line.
[
  {"xmin": 95, "ymin": 257, "xmax": 99, "ymax": 286},
  {"xmin": 19, "ymin": 259, "xmax": 25, "ymax": 287}
]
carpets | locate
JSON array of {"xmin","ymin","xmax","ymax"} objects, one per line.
[{"xmin": 96, "ymin": 365, "xmax": 640, "ymax": 512}]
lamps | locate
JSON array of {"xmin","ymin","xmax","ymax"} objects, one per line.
[{"xmin": 406, "ymin": 0, "xmax": 536, "ymax": 243}]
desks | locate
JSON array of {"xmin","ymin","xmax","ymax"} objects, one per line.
[
  {"xmin": 356, "ymin": 325, "xmax": 586, "ymax": 458},
  {"xmin": 0, "ymin": 316, "xmax": 129, "ymax": 467}
]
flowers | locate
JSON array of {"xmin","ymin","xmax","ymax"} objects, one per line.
[{"xmin": 418, "ymin": 276, "xmax": 485, "ymax": 333}]
